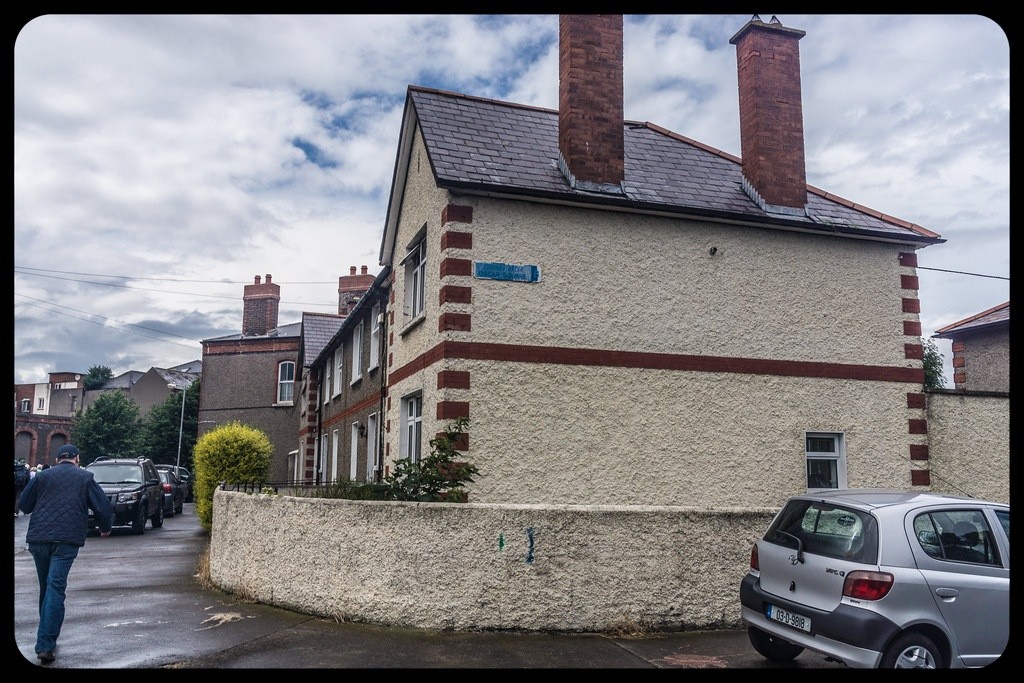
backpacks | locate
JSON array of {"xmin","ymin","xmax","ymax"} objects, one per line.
[{"xmin": 15, "ymin": 464, "xmax": 28, "ymax": 487}]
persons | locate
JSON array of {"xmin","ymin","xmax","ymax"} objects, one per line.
[
  {"xmin": 14, "ymin": 457, "xmax": 50, "ymax": 517},
  {"xmin": 78, "ymin": 455, "xmax": 86, "ymax": 470},
  {"xmin": 19, "ymin": 445, "xmax": 112, "ymax": 661}
]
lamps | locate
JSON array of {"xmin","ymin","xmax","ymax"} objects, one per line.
[{"xmin": 358, "ymin": 423, "xmax": 368, "ymax": 441}]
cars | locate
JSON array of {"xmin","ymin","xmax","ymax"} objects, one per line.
[
  {"xmin": 741, "ymin": 489, "xmax": 1009, "ymax": 668},
  {"xmin": 149, "ymin": 464, "xmax": 192, "ymax": 518}
]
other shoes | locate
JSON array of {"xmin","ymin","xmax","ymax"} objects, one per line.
[
  {"xmin": 37, "ymin": 652, "xmax": 55, "ymax": 661},
  {"xmin": 14, "ymin": 513, "xmax": 18, "ymax": 518}
]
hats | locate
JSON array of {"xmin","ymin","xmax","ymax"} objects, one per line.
[
  {"xmin": 57, "ymin": 444, "xmax": 82, "ymax": 460},
  {"xmin": 37, "ymin": 464, "xmax": 42, "ymax": 468}
]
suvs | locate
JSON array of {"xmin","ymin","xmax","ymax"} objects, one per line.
[{"xmin": 83, "ymin": 455, "xmax": 165, "ymax": 535}]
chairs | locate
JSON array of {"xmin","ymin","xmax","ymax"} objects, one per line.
[{"xmin": 943, "ymin": 522, "xmax": 986, "ymax": 562}]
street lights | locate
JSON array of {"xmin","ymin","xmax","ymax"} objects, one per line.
[{"xmin": 167, "ymin": 383, "xmax": 186, "ymax": 481}]
